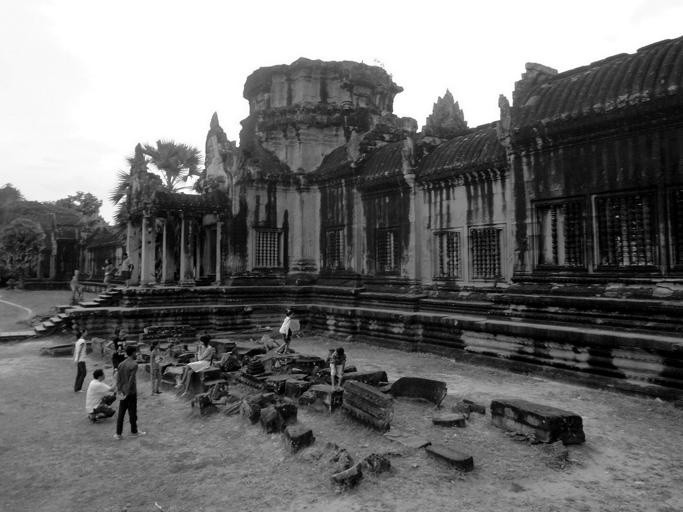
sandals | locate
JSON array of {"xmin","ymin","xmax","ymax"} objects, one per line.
[{"xmin": 174, "ymin": 381, "xmax": 189, "ymax": 398}]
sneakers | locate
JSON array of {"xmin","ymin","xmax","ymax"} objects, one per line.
[
  {"xmin": 87, "ymin": 416, "xmax": 99, "ymax": 423},
  {"xmin": 132, "ymin": 430, "xmax": 146, "ymax": 438},
  {"xmin": 112, "ymin": 432, "xmax": 121, "ymax": 440}
]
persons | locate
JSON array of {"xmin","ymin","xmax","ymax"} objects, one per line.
[
  {"xmin": 99, "ymin": 259, "xmax": 116, "ymax": 291},
  {"xmin": 175, "ymin": 336, "xmax": 213, "ymax": 397},
  {"xmin": 148, "ymin": 342, "xmax": 164, "ymax": 393},
  {"xmin": 112, "ymin": 344, "xmax": 125, "ymax": 367},
  {"xmin": 68, "ymin": 270, "xmax": 81, "ymax": 301},
  {"xmin": 84, "ymin": 368, "xmax": 114, "ymax": 423},
  {"xmin": 72, "ymin": 329, "xmax": 88, "ymax": 392},
  {"xmin": 112, "ymin": 346, "xmax": 147, "ymax": 440},
  {"xmin": 121, "ymin": 252, "xmax": 134, "ymax": 288},
  {"xmin": 111, "ymin": 329, "xmax": 128, "ymax": 356},
  {"xmin": 328, "ymin": 347, "xmax": 346, "ymax": 388},
  {"xmin": 276, "ymin": 309, "xmax": 293, "ymax": 355}
]
